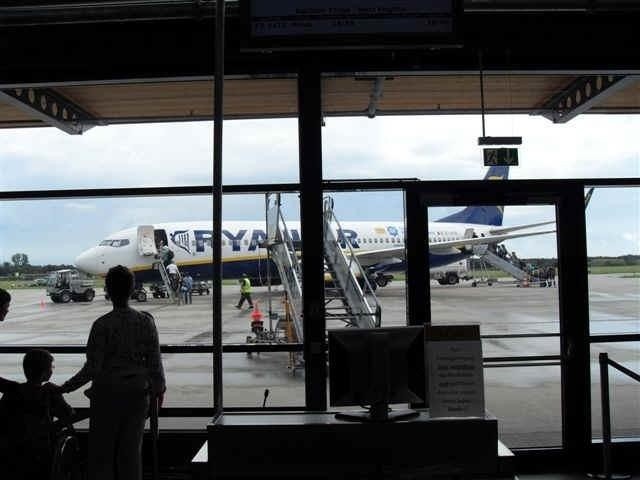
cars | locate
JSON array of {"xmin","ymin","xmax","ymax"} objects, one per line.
[{"xmin": 33, "ymin": 278, "xmax": 48, "ymax": 286}]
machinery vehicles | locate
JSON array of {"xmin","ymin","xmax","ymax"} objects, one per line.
[
  {"xmin": 46, "ymin": 268, "xmax": 96, "ymax": 304},
  {"xmin": 373, "ymin": 271, "xmax": 395, "ymax": 290},
  {"xmin": 425, "ymin": 257, "xmax": 469, "ymax": 287}
]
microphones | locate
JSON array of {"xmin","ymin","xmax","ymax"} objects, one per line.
[{"xmin": 263, "ymin": 389, "xmax": 269, "ymax": 407}]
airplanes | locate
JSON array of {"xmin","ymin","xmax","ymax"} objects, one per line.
[{"xmin": 69, "ymin": 145, "xmax": 597, "ymax": 307}]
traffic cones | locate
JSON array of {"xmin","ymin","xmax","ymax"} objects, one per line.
[{"xmin": 251, "ymin": 302, "xmax": 263, "ymax": 317}]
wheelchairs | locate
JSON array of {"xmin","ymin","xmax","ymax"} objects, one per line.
[{"xmin": 0, "ymin": 398, "xmax": 86, "ymax": 480}]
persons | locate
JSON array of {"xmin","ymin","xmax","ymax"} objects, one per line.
[
  {"xmin": 38, "ymin": 266, "xmax": 166, "ymax": 478},
  {"xmin": 155, "ymin": 237, "xmax": 192, "ymax": 305},
  {"xmin": 234, "ymin": 273, "xmax": 254, "ymax": 309},
  {"xmin": 496, "ymin": 245, "xmax": 556, "ymax": 288},
  {"xmin": 1, "ymin": 350, "xmax": 74, "ymax": 478}
]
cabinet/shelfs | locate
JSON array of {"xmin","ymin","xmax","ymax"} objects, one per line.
[{"xmin": 181, "ymin": 396, "xmax": 519, "ymax": 479}]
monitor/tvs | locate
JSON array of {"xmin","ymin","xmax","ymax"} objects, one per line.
[{"xmin": 328, "ymin": 325, "xmax": 426, "ymax": 422}]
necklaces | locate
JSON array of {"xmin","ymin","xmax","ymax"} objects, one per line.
[{"xmin": 1, "ymin": 288, "xmax": 12, "ymax": 322}]
